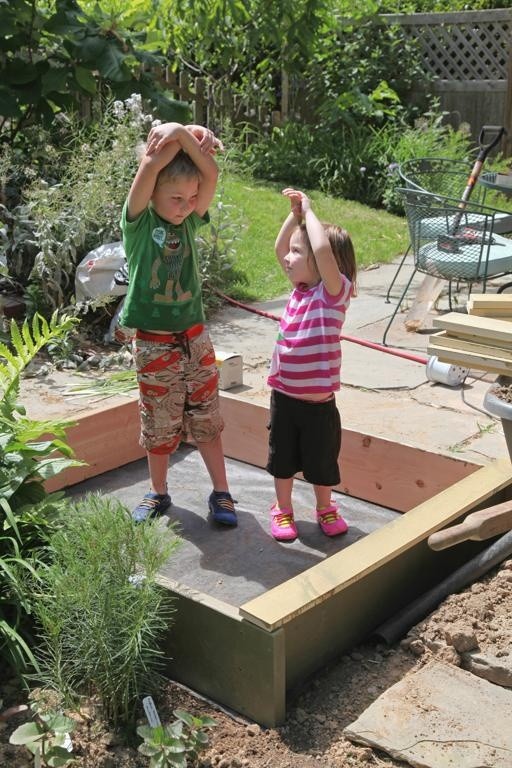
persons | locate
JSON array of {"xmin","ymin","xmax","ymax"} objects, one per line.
[
  {"xmin": 268, "ymin": 187, "xmax": 357, "ymax": 540},
  {"xmin": 117, "ymin": 121, "xmax": 241, "ymax": 527}
]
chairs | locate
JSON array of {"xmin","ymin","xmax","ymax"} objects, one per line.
[{"xmin": 381, "ymin": 156, "xmax": 512, "ymax": 348}]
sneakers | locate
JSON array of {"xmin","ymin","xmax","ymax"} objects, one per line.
[
  {"xmin": 316, "ymin": 499, "xmax": 348, "ymax": 537},
  {"xmin": 208, "ymin": 490, "xmax": 238, "ymax": 526},
  {"xmin": 130, "ymin": 493, "xmax": 172, "ymax": 526},
  {"xmin": 270, "ymin": 503, "xmax": 298, "ymax": 541}
]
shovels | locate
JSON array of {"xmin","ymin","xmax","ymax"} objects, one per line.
[{"xmin": 403, "ymin": 125, "xmax": 504, "ymax": 332}]
